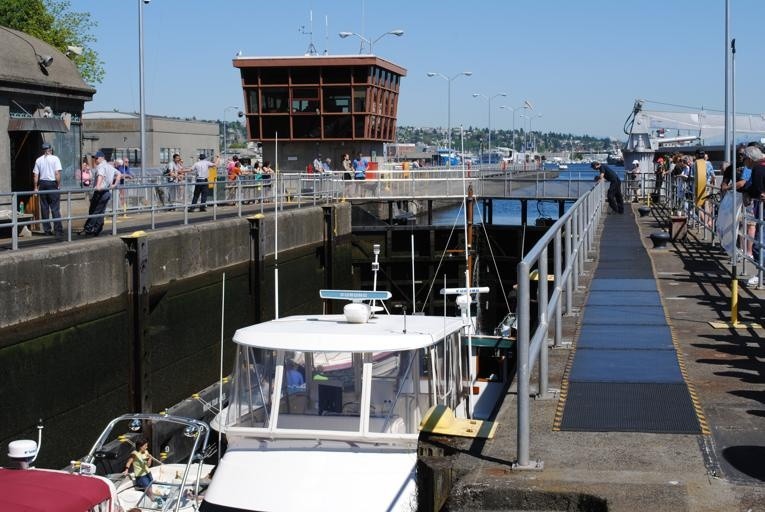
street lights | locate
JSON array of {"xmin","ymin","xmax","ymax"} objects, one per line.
[
  {"xmin": 337, "ymin": 27, "xmax": 406, "ymax": 57},
  {"xmin": 134, "ymin": 1, "xmax": 152, "ymax": 203},
  {"xmin": 470, "ymin": 93, "xmax": 508, "ymax": 167},
  {"xmin": 222, "ymin": 105, "xmax": 245, "ymax": 165},
  {"xmin": 517, "ymin": 113, "xmax": 543, "ymax": 154},
  {"xmin": 427, "ymin": 71, "xmax": 472, "ymax": 169},
  {"xmin": 497, "ymin": 104, "xmax": 529, "ymax": 154}
]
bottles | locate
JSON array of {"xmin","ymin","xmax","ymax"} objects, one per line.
[{"xmin": 19, "ymin": 198, "xmax": 24, "ymax": 216}]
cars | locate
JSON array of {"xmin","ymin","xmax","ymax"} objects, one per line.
[
  {"xmin": 129, "ymin": 166, "xmax": 225, "ymax": 185},
  {"xmin": 462, "ymin": 146, "xmax": 528, "ymax": 167}
]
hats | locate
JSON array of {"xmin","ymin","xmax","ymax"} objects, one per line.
[
  {"xmin": 199, "ymin": 154, "xmax": 206, "ymax": 159},
  {"xmin": 656, "ymin": 158, "xmax": 663, "ymax": 162},
  {"xmin": 632, "ymin": 160, "xmax": 639, "ymax": 164},
  {"xmin": 92, "ymin": 151, "xmax": 104, "ymax": 158},
  {"xmin": 42, "ymin": 143, "xmax": 50, "ymax": 149}
]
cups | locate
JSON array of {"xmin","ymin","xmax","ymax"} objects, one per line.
[{"xmin": 677, "ymin": 211, "xmax": 681, "ymax": 217}]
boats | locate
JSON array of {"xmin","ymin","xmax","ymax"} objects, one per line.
[
  {"xmin": 0, "ymin": 406, "xmax": 215, "ymax": 512},
  {"xmin": 193, "ymin": 319, "xmax": 420, "ymax": 443},
  {"xmin": 546, "ymin": 157, "xmax": 606, "ymax": 171},
  {"xmin": 192, "ymin": 132, "xmax": 514, "ymax": 510}
]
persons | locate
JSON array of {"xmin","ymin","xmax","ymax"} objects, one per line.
[
  {"xmin": 413, "ymin": 158, "xmax": 426, "ymax": 168},
  {"xmin": 627, "ymin": 159, "xmax": 641, "ymax": 203},
  {"xmin": 520, "ymin": 154, "xmax": 546, "ymax": 172},
  {"xmin": 32, "ymin": 143, "xmax": 66, "ymax": 240},
  {"xmin": 74, "ymin": 158, "xmax": 137, "ymax": 218},
  {"xmin": 75, "ymin": 150, "xmax": 122, "ymax": 238},
  {"xmin": 283, "ymin": 362, "xmax": 304, "ymax": 385},
  {"xmin": 162, "ymin": 154, "xmax": 275, "ymax": 212},
  {"xmin": 654, "ymin": 143, "xmax": 765, "ymax": 288},
  {"xmin": 591, "ymin": 162, "xmax": 625, "ymax": 215},
  {"xmin": 307, "ymin": 151, "xmax": 370, "ymax": 197},
  {"xmin": 119, "ymin": 438, "xmax": 155, "ymax": 502}
]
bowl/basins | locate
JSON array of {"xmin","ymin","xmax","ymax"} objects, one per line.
[{"xmin": 343, "ymin": 302, "xmax": 372, "ymax": 322}]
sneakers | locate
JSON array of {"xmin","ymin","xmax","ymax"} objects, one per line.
[{"xmin": 747, "ymin": 276, "xmax": 759, "ymax": 285}]
